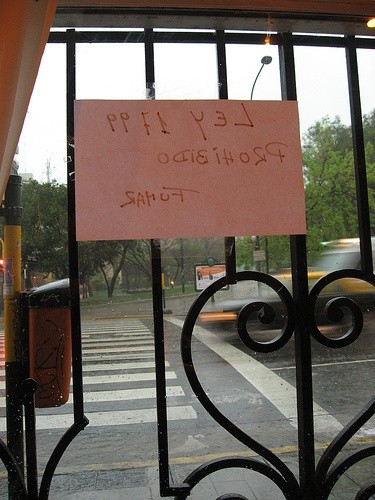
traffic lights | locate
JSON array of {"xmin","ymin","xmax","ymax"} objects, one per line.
[{"xmin": 0, "ymin": 258, "xmax": 4, "ymax": 267}]
